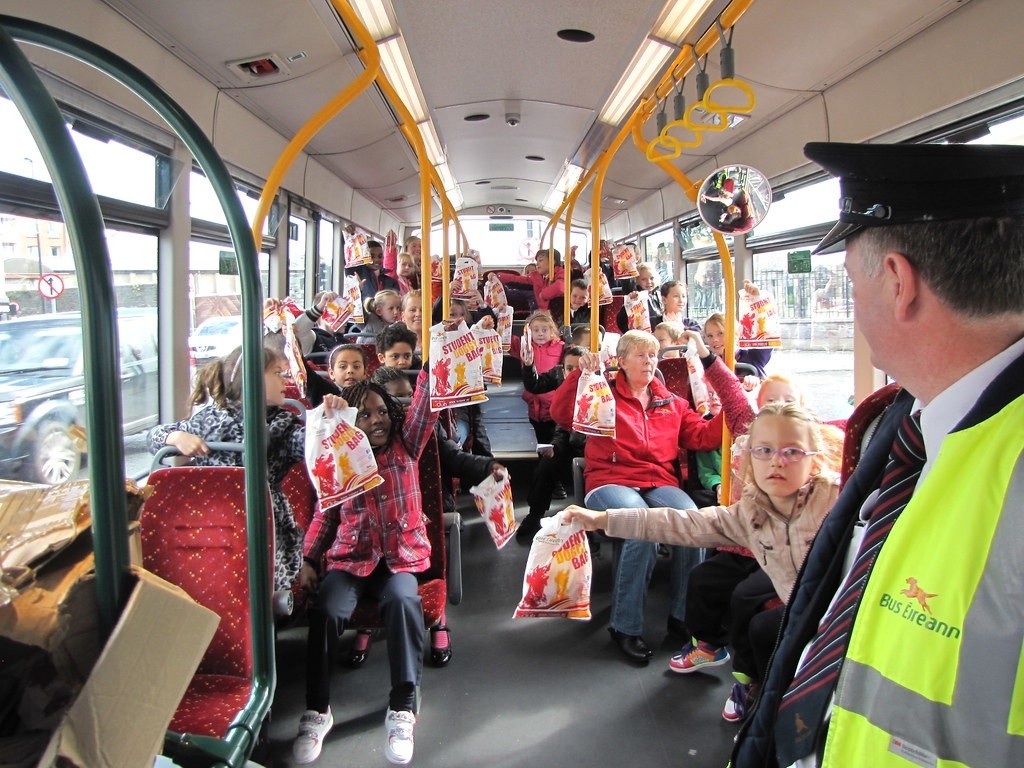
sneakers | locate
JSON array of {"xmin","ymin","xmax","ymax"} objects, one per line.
[
  {"xmin": 383, "ymin": 706, "xmax": 417, "ymax": 765},
  {"xmin": 722, "ymin": 682, "xmax": 757, "ymax": 721},
  {"xmin": 292, "ymin": 706, "xmax": 334, "ymax": 764},
  {"xmin": 667, "ymin": 641, "xmax": 730, "ymax": 673}
]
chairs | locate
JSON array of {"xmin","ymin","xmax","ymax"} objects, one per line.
[{"xmin": 139, "ymin": 251, "xmax": 853, "ymax": 768}]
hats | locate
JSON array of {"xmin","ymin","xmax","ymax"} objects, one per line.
[{"xmin": 804, "ymin": 143, "xmax": 1024, "ymax": 273}]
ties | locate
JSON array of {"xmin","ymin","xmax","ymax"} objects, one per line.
[{"xmin": 770, "ymin": 410, "xmax": 927, "ymax": 768}]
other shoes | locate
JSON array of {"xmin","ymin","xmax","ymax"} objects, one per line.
[
  {"xmin": 514, "ymin": 514, "xmax": 544, "ymax": 544},
  {"xmin": 547, "ymin": 481, "xmax": 567, "ymax": 499}
]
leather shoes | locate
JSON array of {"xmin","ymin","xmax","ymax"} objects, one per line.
[
  {"xmin": 667, "ymin": 616, "xmax": 691, "ymax": 640},
  {"xmin": 609, "ymin": 628, "xmax": 652, "ymax": 661},
  {"xmin": 348, "ymin": 627, "xmax": 370, "ymax": 669},
  {"xmin": 429, "ymin": 625, "xmax": 452, "ymax": 667}
]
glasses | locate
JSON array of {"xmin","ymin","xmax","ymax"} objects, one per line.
[{"xmin": 749, "ymin": 444, "xmax": 822, "ymax": 462}]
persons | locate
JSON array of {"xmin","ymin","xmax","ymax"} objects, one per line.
[
  {"xmin": 564, "ymin": 401, "xmax": 840, "ymax": 607},
  {"xmin": 733, "ymin": 141, "xmax": 1024, "ymax": 768},
  {"xmin": 290, "ymin": 319, "xmax": 459, "ymax": 761},
  {"xmin": 145, "ymin": 344, "xmax": 349, "ymax": 595},
  {"xmin": 267, "ymin": 230, "xmax": 845, "ymax": 723}
]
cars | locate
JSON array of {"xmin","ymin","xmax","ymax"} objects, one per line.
[
  {"xmin": 0, "ymin": 308, "xmax": 198, "ymax": 487},
  {"xmin": 181, "ymin": 313, "xmax": 248, "ymax": 374}
]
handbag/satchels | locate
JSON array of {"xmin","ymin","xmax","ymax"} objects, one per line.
[
  {"xmin": 342, "ymin": 230, "xmax": 373, "ymax": 269},
  {"xmin": 584, "ymin": 265, "xmax": 613, "ymax": 308},
  {"xmin": 623, "ymin": 290, "xmax": 653, "ymax": 332},
  {"xmin": 684, "ymin": 339, "xmax": 710, "ymax": 411},
  {"xmin": 469, "ymin": 467, "xmax": 518, "ymax": 551},
  {"xmin": 511, "ymin": 515, "xmax": 593, "ymax": 623},
  {"xmin": 573, "ymin": 361, "xmax": 615, "ymax": 436},
  {"xmin": 700, "ymin": 195, "xmax": 707, "ymax": 204},
  {"xmin": 613, "ymin": 245, "xmax": 639, "ymax": 280},
  {"xmin": 738, "ymin": 288, "xmax": 784, "ymax": 348},
  {"xmin": 263, "ymin": 272, "xmax": 364, "ymax": 398},
  {"xmin": 306, "ymin": 402, "xmax": 387, "ymax": 514},
  {"xmin": 429, "ymin": 251, "xmax": 513, "ymax": 412}
]
